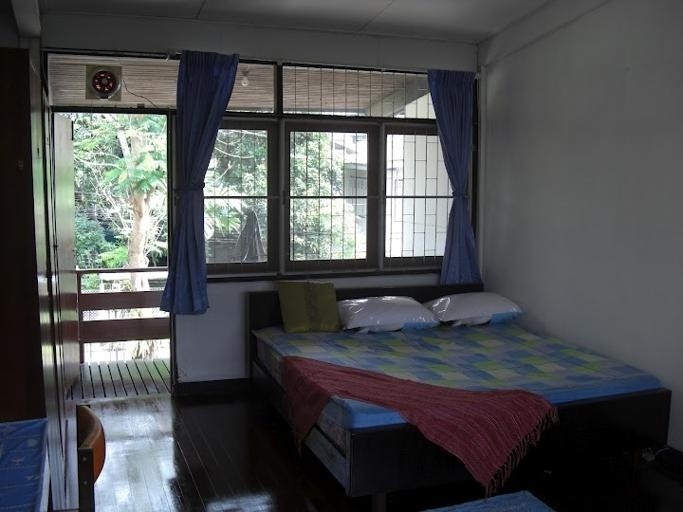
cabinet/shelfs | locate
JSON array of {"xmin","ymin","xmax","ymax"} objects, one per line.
[{"xmin": 0, "ymin": 48, "xmax": 68, "ymax": 512}]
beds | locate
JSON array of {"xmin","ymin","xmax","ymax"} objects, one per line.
[{"xmin": 245, "ymin": 284, "xmax": 672, "ymax": 512}]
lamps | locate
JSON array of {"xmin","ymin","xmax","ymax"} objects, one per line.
[{"xmin": 241, "ymin": 71, "xmax": 250, "ymax": 87}]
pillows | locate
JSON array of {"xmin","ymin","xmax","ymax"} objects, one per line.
[
  {"xmin": 423, "ymin": 292, "xmax": 525, "ymax": 328},
  {"xmin": 277, "ymin": 281, "xmax": 343, "ymax": 332},
  {"xmin": 337, "ymin": 295, "xmax": 440, "ymax": 333}
]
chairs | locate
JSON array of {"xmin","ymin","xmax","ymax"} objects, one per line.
[{"xmin": 54, "ymin": 404, "xmax": 106, "ymax": 512}]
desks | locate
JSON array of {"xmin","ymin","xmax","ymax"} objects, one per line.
[{"xmin": 1, "ymin": 418, "xmax": 51, "ymax": 512}]
